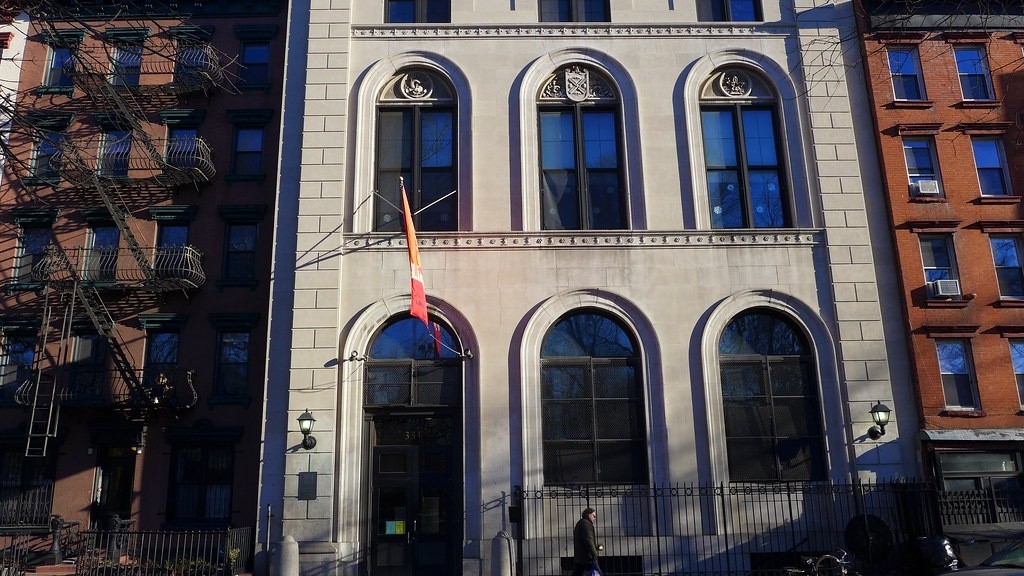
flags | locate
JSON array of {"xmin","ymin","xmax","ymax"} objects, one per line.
[
  {"xmin": 431, "ymin": 322, "xmax": 441, "ymax": 359},
  {"xmin": 400, "ymin": 183, "xmax": 428, "ymax": 330}
]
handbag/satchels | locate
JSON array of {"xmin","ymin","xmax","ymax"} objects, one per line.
[{"xmin": 586, "ymin": 560, "xmax": 601, "ymax": 576}]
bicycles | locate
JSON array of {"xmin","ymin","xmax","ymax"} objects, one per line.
[{"xmin": 781, "ymin": 547, "xmax": 864, "ymax": 576}]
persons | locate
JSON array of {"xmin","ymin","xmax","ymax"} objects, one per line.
[{"xmin": 570, "ymin": 508, "xmax": 602, "ymax": 576}]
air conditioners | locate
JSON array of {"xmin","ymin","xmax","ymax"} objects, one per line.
[
  {"xmin": 929, "ymin": 280, "xmax": 962, "ymax": 299},
  {"xmin": 913, "ymin": 179, "xmax": 939, "ymax": 197}
]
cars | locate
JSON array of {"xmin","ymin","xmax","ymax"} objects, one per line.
[{"xmin": 934, "ymin": 535, "xmax": 1024, "ymax": 576}]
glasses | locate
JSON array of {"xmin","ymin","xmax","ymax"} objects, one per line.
[{"xmin": 591, "ymin": 513, "xmax": 596, "ymax": 518}]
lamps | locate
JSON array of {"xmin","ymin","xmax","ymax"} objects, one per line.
[
  {"xmin": 866, "ymin": 400, "xmax": 893, "ymax": 440},
  {"xmin": 348, "ymin": 351, "xmax": 359, "ymax": 361},
  {"xmin": 463, "ymin": 348, "xmax": 474, "ymax": 360},
  {"xmin": 297, "ymin": 408, "xmax": 317, "ymax": 449}
]
